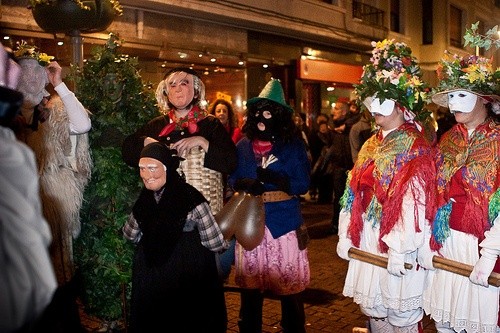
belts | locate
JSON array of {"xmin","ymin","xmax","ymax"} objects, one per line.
[{"xmin": 260, "ymin": 190, "xmax": 297, "ymax": 203}]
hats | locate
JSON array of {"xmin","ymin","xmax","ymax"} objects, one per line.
[
  {"xmin": 247, "ymin": 80, "xmax": 294, "ymax": 115},
  {"xmin": 431, "ymin": 56, "xmax": 499, "ymax": 108},
  {"xmin": 352, "ymin": 39, "xmax": 433, "ymax": 132}
]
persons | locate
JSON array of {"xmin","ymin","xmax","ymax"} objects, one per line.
[
  {"xmin": 291, "ymin": 111, "xmax": 333, "ymax": 202},
  {"xmin": 337, "ymin": 102, "xmax": 435, "ymax": 333},
  {"xmin": 11, "ymin": 45, "xmax": 92, "ymax": 333},
  {"xmin": 102, "ymin": 73, "xmax": 125, "ymax": 111},
  {"xmin": 122, "ymin": 141, "xmax": 228, "ymax": 333},
  {"xmin": 429, "ymin": 92, "xmax": 500, "ymax": 333},
  {"xmin": 0, "ymin": 45, "xmax": 58, "ymax": 333},
  {"xmin": 123, "ymin": 65, "xmax": 234, "ymax": 286},
  {"xmin": 210, "ymin": 94, "xmax": 236, "ymax": 135},
  {"xmin": 435, "ymin": 106, "xmax": 452, "ymax": 143},
  {"xmin": 325, "ymin": 99, "xmax": 372, "ymax": 234},
  {"xmin": 224, "ymin": 98, "xmax": 319, "ymax": 333}
]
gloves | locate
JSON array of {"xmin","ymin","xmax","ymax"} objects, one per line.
[
  {"xmin": 386, "ymin": 248, "xmax": 408, "ymax": 277},
  {"xmin": 417, "ymin": 244, "xmax": 442, "ymax": 270},
  {"xmin": 336, "ymin": 235, "xmax": 357, "ymax": 262},
  {"xmin": 468, "ymin": 249, "xmax": 498, "ymax": 287}
]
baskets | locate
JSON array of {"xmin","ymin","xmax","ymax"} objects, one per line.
[{"xmin": 176, "ymin": 148, "xmax": 224, "ymax": 216}]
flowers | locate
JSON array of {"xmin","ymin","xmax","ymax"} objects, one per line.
[
  {"xmin": 346, "ymin": 36, "xmax": 434, "ymax": 120},
  {"xmin": 436, "ymin": 21, "xmax": 500, "ymax": 97}
]
